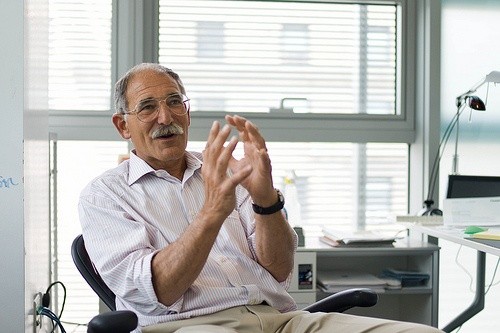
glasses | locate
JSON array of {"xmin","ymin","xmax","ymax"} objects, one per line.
[{"xmin": 122, "ymin": 94, "xmax": 191, "ymax": 123}]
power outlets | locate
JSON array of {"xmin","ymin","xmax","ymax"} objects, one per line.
[
  {"xmin": 40, "ymin": 291, "xmax": 50, "ymax": 319},
  {"xmin": 33, "ymin": 293, "xmax": 41, "ymax": 333}
]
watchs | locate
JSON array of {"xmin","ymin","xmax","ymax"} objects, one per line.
[{"xmin": 251, "ymin": 188, "xmax": 285, "ymax": 215}]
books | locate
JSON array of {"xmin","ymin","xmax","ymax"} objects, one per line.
[{"xmin": 318, "ymin": 224, "xmax": 396, "ymax": 248}]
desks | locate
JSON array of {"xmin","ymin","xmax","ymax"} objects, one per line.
[{"xmin": 422, "ymin": 223, "xmax": 500, "ymax": 333}]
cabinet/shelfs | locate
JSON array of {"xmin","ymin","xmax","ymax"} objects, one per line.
[{"xmin": 289, "ymin": 237, "xmax": 442, "ymax": 329}]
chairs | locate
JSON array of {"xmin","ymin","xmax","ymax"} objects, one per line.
[{"xmin": 70, "ymin": 234, "xmax": 378, "ymax": 333}]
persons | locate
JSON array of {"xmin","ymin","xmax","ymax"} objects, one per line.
[{"xmin": 78, "ymin": 63, "xmax": 450, "ymax": 333}]
